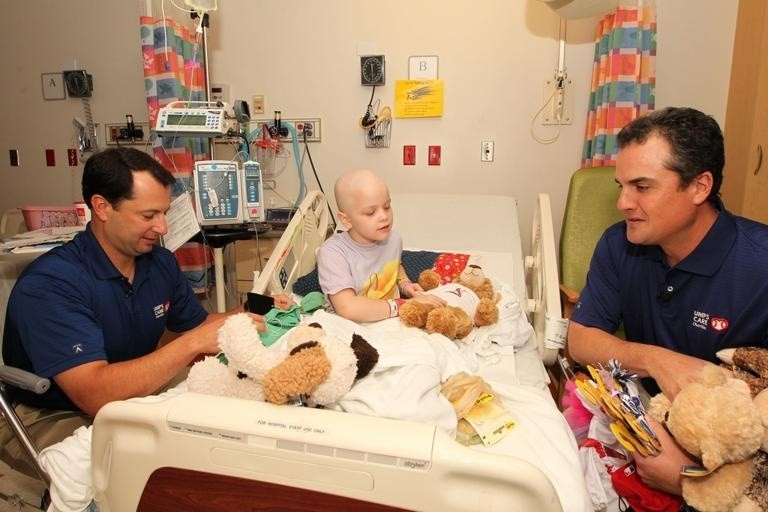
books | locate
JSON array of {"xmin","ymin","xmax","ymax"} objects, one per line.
[{"xmin": 12, "ymin": 242, "xmax": 66, "ymax": 254}]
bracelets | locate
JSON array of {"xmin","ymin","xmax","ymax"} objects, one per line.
[{"xmin": 387, "ymin": 296, "xmax": 406, "ymax": 319}]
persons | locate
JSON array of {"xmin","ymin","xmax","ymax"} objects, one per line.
[
  {"xmin": 1, "ymin": 145, "xmax": 298, "ymax": 511},
  {"xmin": 317, "ymin": 168, "xmax": 449, "ymax": 325},
  {"xmin": 565, "ymin": 104, "xmax": 767, "ymax": 495}
]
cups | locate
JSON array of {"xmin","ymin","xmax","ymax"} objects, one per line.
[{"xmin": 73, "ymin": 200, "xmax": 91, "ymax": 225}]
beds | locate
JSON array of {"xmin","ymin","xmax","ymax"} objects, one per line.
[{"xmin": 92, "ymin": 190, "xmax": 592, "ymax": 510}]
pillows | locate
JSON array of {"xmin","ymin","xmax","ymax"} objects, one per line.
[{"xmin": 293, "ymin": 249, "xmax": 470, "ymax": 299}]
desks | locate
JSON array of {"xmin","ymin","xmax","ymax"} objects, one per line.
[{"xmin": 186, "ymin": 229, "xmax": 251, "ymax": 314}]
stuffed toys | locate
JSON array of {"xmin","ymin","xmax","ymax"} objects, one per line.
[
  {"xmin": 184, "ymin": 312, "xmax": 380, "ymax": 409},
  {"xmin": 649, "ymin": 345, "xmax": 767, "ymax": 512},
  {"xmin": 398, "ymin": 264, "xmax": 503, "ymax": 341}
]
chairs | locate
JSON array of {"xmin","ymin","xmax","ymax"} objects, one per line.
[
  {"xmin": 546, "ymin": 163, "xmax": 627, "ymax": 412},
  {"xmin": 0, "ymin": 253, "xmax": 52, "ymax": 512}
]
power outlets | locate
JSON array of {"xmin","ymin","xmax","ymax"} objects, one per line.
[
  {"xmin": 245, "ymin": 118, "xmax": 320, "ymax": 142},
  {"xmin": 481, "ymin": 141, "xmax": 494, "ymax": 161}
]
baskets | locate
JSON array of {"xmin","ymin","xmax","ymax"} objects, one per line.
[{"xmin": 17, "ymin": 204, "xmax": 79, "ymax": 232}]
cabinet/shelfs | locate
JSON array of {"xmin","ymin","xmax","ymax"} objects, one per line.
[{"xmin": 231, "ymin": 235, "xmax": 287, "ymax": 309}]
